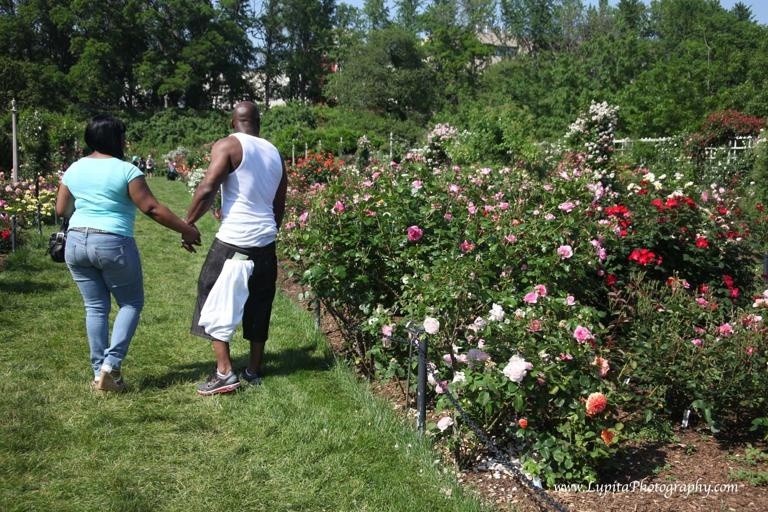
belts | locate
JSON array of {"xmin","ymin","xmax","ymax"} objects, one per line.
[{"xmin": 68, "ymin": 227, "xmax": 119, "ymax": 235}]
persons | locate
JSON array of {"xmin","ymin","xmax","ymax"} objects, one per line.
[
  {"xmin": 123, "ymin": 152, "xmax": 178, "ymax": 181},
  {"xmin": 55, "ymin": 115, "xmax": 202, "ymax": 394},
  {"xmin": 181, "ymin": 102, "xmax": 288, "ymax": 396}
]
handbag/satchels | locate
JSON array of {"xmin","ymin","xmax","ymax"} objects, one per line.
[{"xmin": 45, "ymin": 218, "xmax": 68, "ymax": 262}]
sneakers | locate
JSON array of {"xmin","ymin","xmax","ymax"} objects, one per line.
[
  {"xmin": 235, "ymin": 365, "xmax": 261, "ymax": 386},
  {"xmin": 98, "ymin": 365, "xmax": 131, "ymax": 393},
  {"xmin": 196, "ymin": 368, "xmax": 241, "ymax": 395}
]
